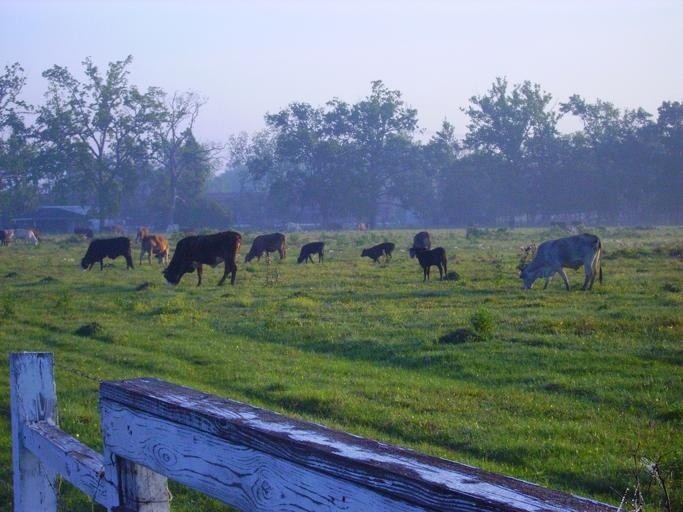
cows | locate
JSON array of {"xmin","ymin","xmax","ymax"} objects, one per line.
[
  {"xmin": 111, "ymin": 223, "xmax": 127, "ymax": 237},
  {"xmin": 160, "ymin": 231, "xmax": 241, "ymax": 288},
  {"xmin": 0, "ymin": 226, "xmax": 40, "ymax": 248},
  {"xmin": 296, "ymin": 240, "xmax": 325, "ymax": 265},
  {"xmin": 360, "ymin": 242, "xmax": 395, "ymax": 264},
  {"xmin": 138, "ymin": 234, "xmax": 169, "ymax": 267},
  {"xmin": 406, "ymin": 229, "xmax": 448, "ymax": 282},
  {"xmin": 244, "ymin": 231, "xmax": 285, "ymax": 264},
  {"xmin": 516, "ymin": 233, "xmax": 602, "ymax": 291},
  {"xmin": 80, "ymin": 236, "xmax": 133, "ymax": 273},
  {"xmin": 74, "ymin": 224, "xmax": 92, "ymax": 240},
  {"xmin": 135, "ymin": 226, "xmax": 148, "ymax": 245}
]
